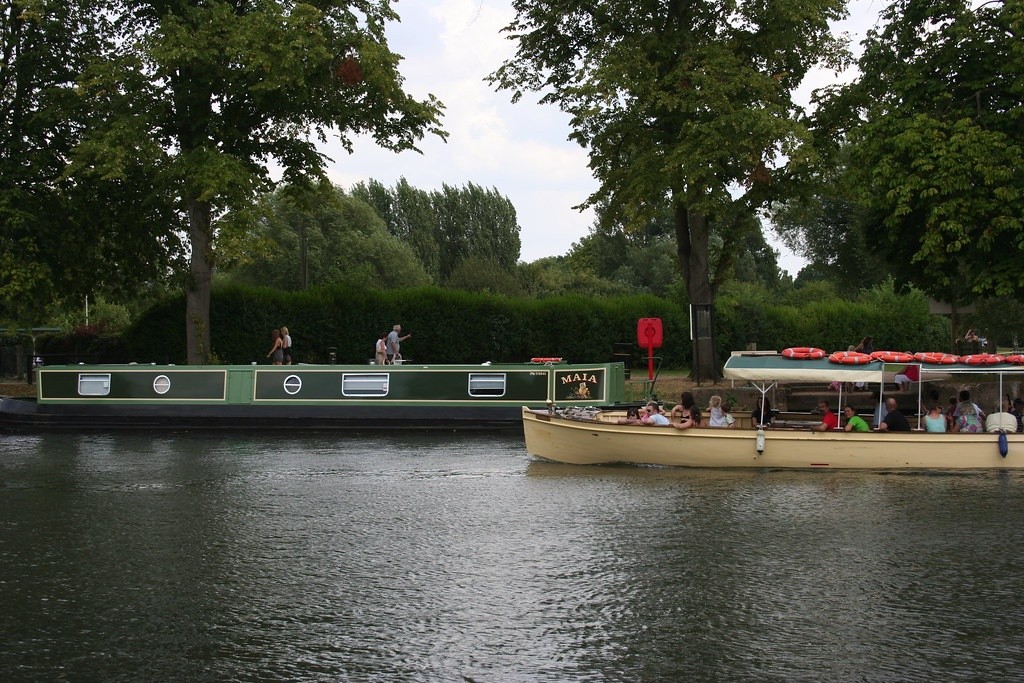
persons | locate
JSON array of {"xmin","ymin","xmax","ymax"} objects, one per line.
[
  {"xmin": 895, "ymin": 351, "xmax": 919, "ymax": 393},
  {"xmin": 375, "ymin": 325, "xmax": 411, "ymax": 365},
  {"xmin": 828, "ymin": 335, "xmax": 873, "ymax": 391},
  {"xmin": 751, "ymin": 397, "xmax": 776, "ymax": 428},
  {"xmin": 266, "ymin": 327, "xmax": 293, "ymax": 364},
  {"xmin": 1010, "ymin": 399, "xmax": 1024, "ymax": 432},
  {"xmin": 617, "ymin": 392, "xmax": 736, "ymax": 429},
  {"xmin": 965, "ymin": 330, "xmax": 978, "ymax": 342},
  {"xmin": 809, "ymin": 390, "xmax": 987, "ymax": 432}
]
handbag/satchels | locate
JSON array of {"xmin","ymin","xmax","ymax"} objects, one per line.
[
  {"xmin": 283, "ymin": 349, "xmax": 291, "ymax": 363},
  {"xmin": 391, "ymin": 353, "xmax": 402, "ymax": 364}
]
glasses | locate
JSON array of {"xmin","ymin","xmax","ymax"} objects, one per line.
[
  {"xmin": 628, "ymin": 414, "xmax": 635, "ymax": 417},
  {"xmin": 646, "ymin": 410, "xmax": 654, "ymax": 413}
]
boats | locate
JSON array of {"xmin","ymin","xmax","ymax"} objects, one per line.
[
  {"xmin": 520, "ymin": 347, "xmax": 1024, "ymax": 471},
  {"xmin": 0, "ymin": 347, "xmax": 677, "ymax": 431}
]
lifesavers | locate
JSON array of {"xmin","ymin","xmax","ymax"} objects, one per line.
[
  {"xmin": 1006, "ymin": 354, "xmax": 1024, "ymax": 365},
  {"xmin": 959, "ymin": 354, "xmax": 1006, "ymax": 365},
  {"xmin": 871, "ymin": 351, "xmax": 914, "ymax": 363},
  {"xmin": 830, "ymin": 352, "xmax": 872, "ymax": 364},
  {"xmin": 782, "ymin": 347, "xmax": 825, "ymax": 360},
  {"xmin": 914, "ymin": 351, "xmax": 960, "ymax": 364}
]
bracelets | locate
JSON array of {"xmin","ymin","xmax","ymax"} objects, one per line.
[{"xmin": 811, "ymin": 425, "xmax": 815, "ymax": 429}]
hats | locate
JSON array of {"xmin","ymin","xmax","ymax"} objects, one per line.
[{"xmin": 393, "ymin": 325, "xmax": 401, "ymax": 331}]
